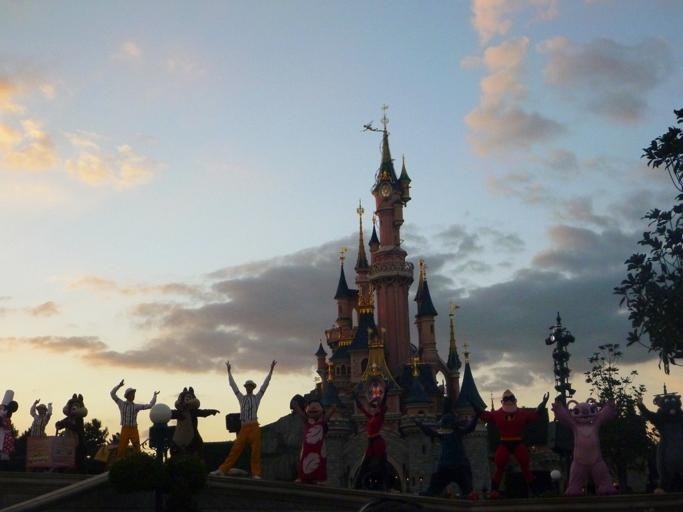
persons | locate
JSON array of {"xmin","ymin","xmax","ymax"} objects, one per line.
[
  {"xmin": 209, "ymin": 360, "xmax": 277, "ymax": 480},
  {"xmin": 468, "ymin": 389, "xmax": 549, "ymax": 501},
  {"xmin": 110, "ymin": 379, "xmax": 160, "ymax": 460},
  {"xmin": 30, "ymin": 399, "xmax": 52, "ymax": 472},
  {"xmin": 352, "ymin": 388, "xmax": 391, "ymax": 494}
]
